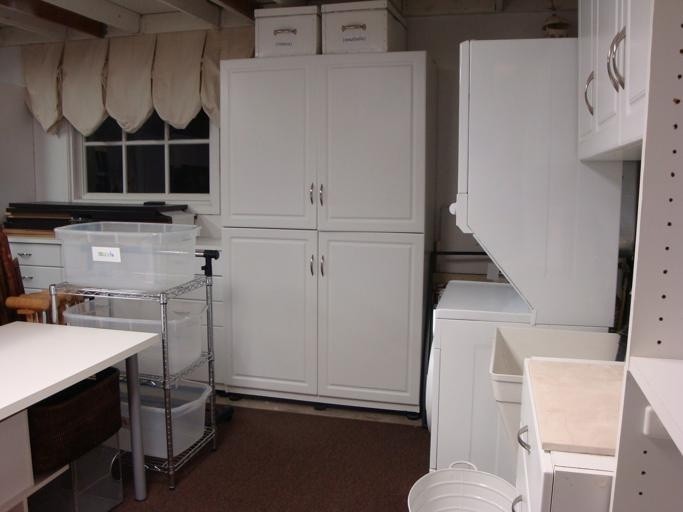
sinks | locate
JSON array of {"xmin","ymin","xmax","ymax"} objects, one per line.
[{"xmin": 488, "ymin": 325, "xmax": 627, "ymax": 448}]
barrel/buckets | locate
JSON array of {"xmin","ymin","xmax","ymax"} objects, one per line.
[{"xmin": 408, "ymin": 461, "xmax": 522, "ymax": 512}]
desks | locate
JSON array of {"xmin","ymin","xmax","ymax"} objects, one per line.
[{"xmin": 0, "ymin": 317, "xmax": 159, "ymax": 512}]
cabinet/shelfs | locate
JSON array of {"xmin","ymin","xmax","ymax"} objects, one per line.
[
  {"xmin": 215, "ymin": 229, "xmax": 434, "ymax": 413},
  {"xmin": 578, "ymin": 0, "xmax": 654, "ymax": 161},
  {"xmin": 8, "ymin": 236, "xmax": 63, "ymax": 322},
  {"xmin": 448, "ymin": 38, "xmax": 625, "ymax": 319},
  {"xmin": 509, "ymin": 357, "xmax": 625, "ymax": 512},
  {"xmin": 219, "ymin": 51, "xmax": 437, "ymax": 232},
  {"xmin": 610, "ymin": 0, "xmax": 683, "ymax": 512},
  {"xmin": 49, "ymin": 249, "xmax": 220, "ymax": 489}
]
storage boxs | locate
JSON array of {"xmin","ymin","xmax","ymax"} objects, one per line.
[
  {"xmin": 54, "ymin": 220, "xmax": 203, "ymax": 292},
  {"xmin": 28, "ymin": 368, "xmax": 122, "ymax": 472},
  {"xmin": 254, "ymin": 5, "xmax": 321, "ymax": 58},
  {"xmin": 104, "ymin": 381, "xmax": 213, "ymax": 459},
  {"xmin": 318, "ymin": 0, "xmax": 407, "ymax": 54},
  {"xmin": 61, "ymin": 300, "xmax": 209, "ymax": 377}
]
